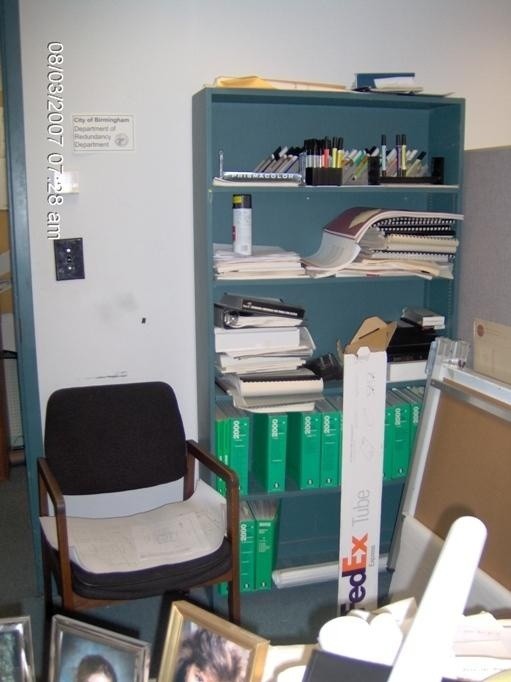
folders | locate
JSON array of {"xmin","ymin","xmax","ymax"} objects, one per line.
[
  {"xmin": 213, "ymin": 302, "xmax": 308, "ymax": 329},
  {"xmin": 220, "ymin": 291, "xmax": 305, "ymax": 320}
]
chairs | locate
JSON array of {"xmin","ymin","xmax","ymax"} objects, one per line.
[{"xmin": 37, "ymin": 379, "xmax": 248, "ymax": 648}]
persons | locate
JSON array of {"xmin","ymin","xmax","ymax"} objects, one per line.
[
  {"xmin": 172, "ymin": 627, "xmax": 242, "ymax": 682},
  {"xmin": 68, "ymin": 653, "xmax": 120, "ymax": 682}
]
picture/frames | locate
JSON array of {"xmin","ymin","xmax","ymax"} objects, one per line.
[{"xmin": 0, "ymin": 599, "xmax": 270, "ymax": 681}]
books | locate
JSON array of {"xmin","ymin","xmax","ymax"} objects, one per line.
[
  {"xmin": 212, "ymin": 293, "xmax": 326, "ymax": 413},
  {"xmin": 214, "ymin": 243, "xmax": 308, "ymax": 281},
  {"xmin": 306, "ymin": 208, "xmax": 464, "ymax": 280}
]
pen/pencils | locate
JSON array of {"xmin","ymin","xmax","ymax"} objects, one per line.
[
  {"xmin": 382, "ymin": 134, "xmax": 387, "ymax": 177},
  {"xmin": 396, "ymin": 134, "xmax": 401, "ymax": 176},
  {"xmin": 305, "ymin": 135, "xmax": 344, "ymax": 168},
  {"xmin": 402, "ymin": 134, "xmax": 406, "ymax": 176},
  {"xmin": 405, "ymin": 151, "xmax": 426, "ymax": 176}
]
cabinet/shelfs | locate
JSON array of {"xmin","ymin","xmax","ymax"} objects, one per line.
[{"xmin": 188, "ymin": 86, "xmax": 469, "ymax": 620}]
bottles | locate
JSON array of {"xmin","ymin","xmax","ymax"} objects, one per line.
[{"xmin": 232, "ymin": 193, "xmax": 254, "ymax": 255}]
